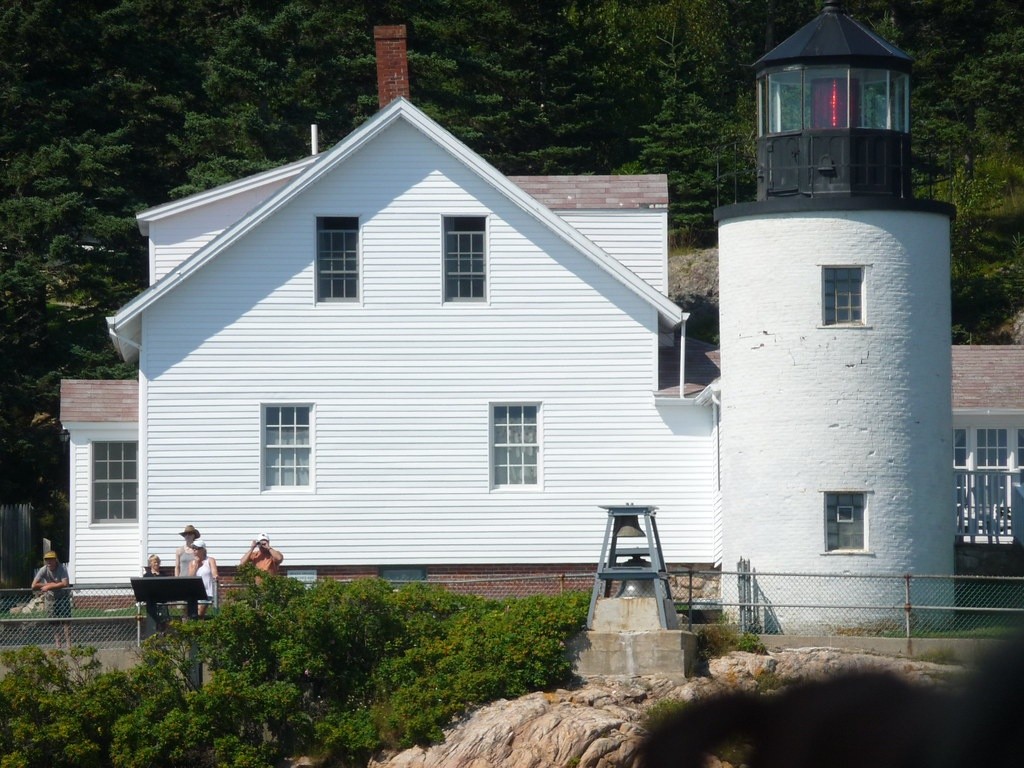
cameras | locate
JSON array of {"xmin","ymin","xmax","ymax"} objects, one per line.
[{"xmin": 256, "ymin": 540, "xmax": 261, "ymax": 545}]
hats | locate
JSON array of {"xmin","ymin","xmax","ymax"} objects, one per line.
[
  {"xmin": 255, "ymin": 533, "xmax": 269, "ymax": 544},
  {"xmin": 190, "ymin": 541, "xmax": 206, "ymax": 549},
  {"xmin": 43, "ymin": 551, "xmax": 57, "ymax": 559},
  {"xmin": 179, "ymin": 525, "xmax": 200, "ymax": 540}
]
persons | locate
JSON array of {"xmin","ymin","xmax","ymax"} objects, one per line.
[
  {"xmin": 240, "ymin": 534, "xmax": 284, "ymax": 586},
  {"xmin": 173, "ymin": 524, "xmax": 202, "ymax": 624},
  {"xmin": 31, "ymin": 551, "xmax": 71, "ymax": 651},
  {"xmin": 141, "ymin": 554, "xmax": 172, "ymax": 640},
  {"xmin": 187, "ymin": 540, "xmax": 220, "ymax": 620}
]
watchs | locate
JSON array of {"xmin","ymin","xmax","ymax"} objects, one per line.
[{"xmin": 267, "ymin": 547, "xmax": 272, "ymax": 553}]
